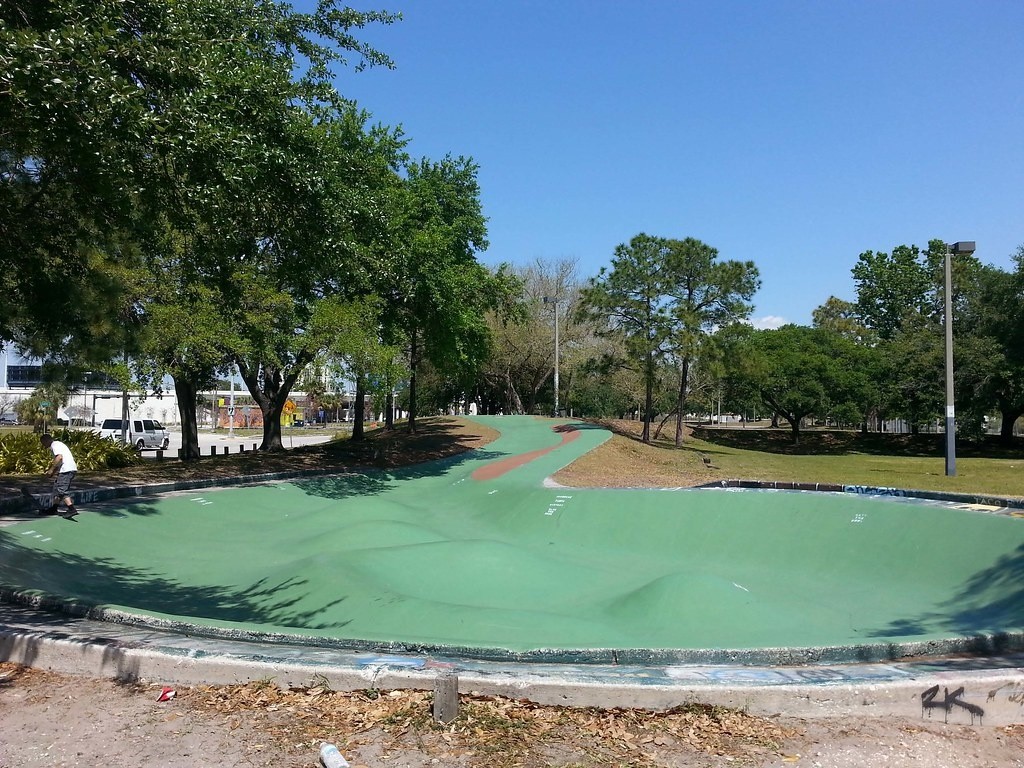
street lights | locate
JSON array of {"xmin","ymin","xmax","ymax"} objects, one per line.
[
  {"xmin": 942, "ymin": 239, "xmax": 977, "ymax": 479},
  {"xmin": 542, "ymin": 296, "xmax": 561, "ymax": 417}
]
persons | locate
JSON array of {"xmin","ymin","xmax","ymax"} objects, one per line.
[{"xmin": 41, "ymin": 434, "xmax": 80, "ymax": 518}]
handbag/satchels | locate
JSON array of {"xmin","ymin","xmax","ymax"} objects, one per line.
[{"xmin": 17, "ymin": 488, "xmax": 43, "ymax": 516}]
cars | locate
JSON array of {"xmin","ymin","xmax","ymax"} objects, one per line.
[{"xmin": 0, "ymin": 418, "xmax": 19, "ymax": 425}]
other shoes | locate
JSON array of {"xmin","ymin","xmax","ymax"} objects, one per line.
[
  {"xmin": 43, "ymin": 508, "xmax": 57, "ymax": 515},
  {"xmin": 63, "ymin": 509, "xmax": 77, "ymax": 519}
]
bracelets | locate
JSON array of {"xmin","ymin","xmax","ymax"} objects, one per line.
[{"xmin": 52, "ymin": 461, "xmax": 58, "ymax": 467}]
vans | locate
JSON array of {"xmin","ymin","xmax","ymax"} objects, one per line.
[{"xmin": 100, "ymin": 417, "xmax": 171, "ymax": 451}]
